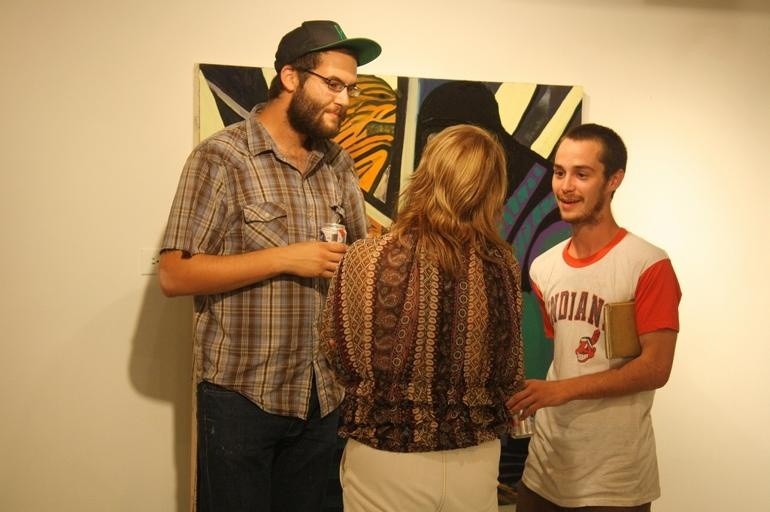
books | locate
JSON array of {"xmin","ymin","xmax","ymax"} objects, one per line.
[{"xmin": 603, "ymin": 300, "xmax": 643, "ymax": 360}]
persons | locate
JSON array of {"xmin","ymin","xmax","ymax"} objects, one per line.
[
  {"xmin": 504, "ymin": 121, "xmax": 683, "ymax": 512},
  {"xmin": 318, "ymin": 120, "xmax": 529, "ymax": 512},
  {"xmin": 153, "ymin": 18, "xmax": 383, "ymax": 512}
]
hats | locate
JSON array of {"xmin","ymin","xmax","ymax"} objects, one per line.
[{"xmin": 273, "ymin": 20, "xmax": 381, "ymax": 67}]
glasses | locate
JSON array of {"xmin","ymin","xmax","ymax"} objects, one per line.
[{"xmin": 303, "ymin": 67, "xmax": 360, "ymax": 97}]
